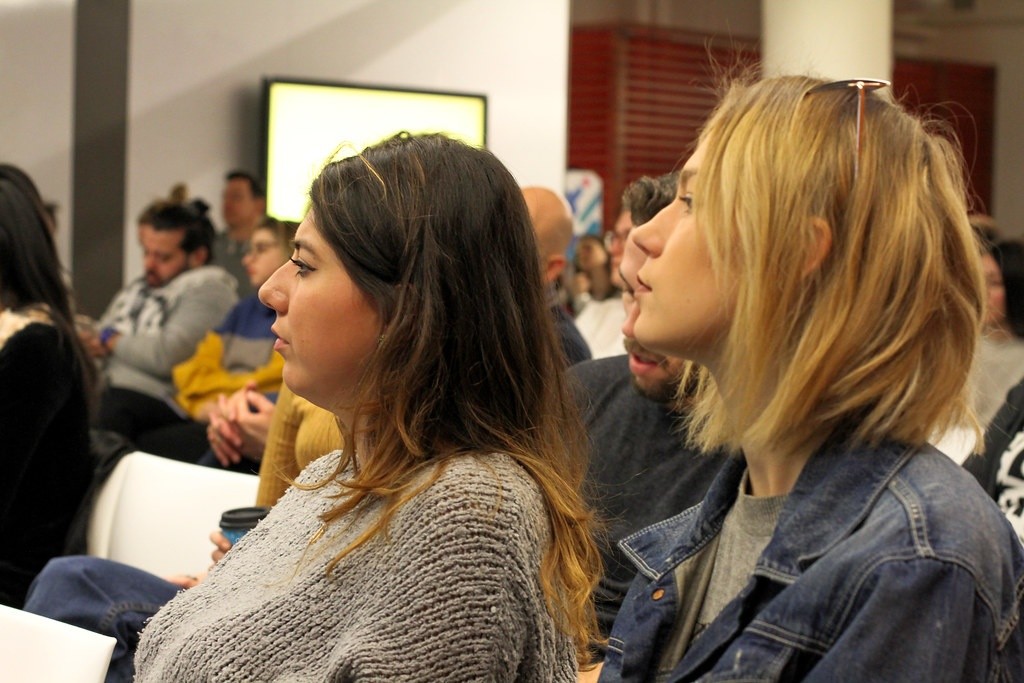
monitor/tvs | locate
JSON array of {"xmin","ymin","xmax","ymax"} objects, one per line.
[{"xmin": 254, "ymin": 75, "xmax": 490, "ymax": 232}]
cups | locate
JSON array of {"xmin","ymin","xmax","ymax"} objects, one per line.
[{"xmin": 219, "ymin": 508, "xmax": 272, "ymax": 544}]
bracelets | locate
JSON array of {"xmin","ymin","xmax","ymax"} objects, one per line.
[{"xmin": 101, "ymin": 327, "xmax": 115, "ymax": 341}]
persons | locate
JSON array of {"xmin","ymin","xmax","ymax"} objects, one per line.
[
  {"xmin": 0, "ymin": 164, "xmax": 98, "ymax": 609},
  {"xmin": 134, "ymin": 132, "xmax": 611, "ymax": 683},
  {"xmin": 23, "ymin": 42, "xmax": 1024, "ymax": 683}
]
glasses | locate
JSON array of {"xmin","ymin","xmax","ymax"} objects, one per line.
[
  {"xmin": 808, "ymin": 77, "xmax": 889, "ymax": 185},
  {"xmin": 602, "ymin": 229, "xmax": 633, "ymax": 256}
]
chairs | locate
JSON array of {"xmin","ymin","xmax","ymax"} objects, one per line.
[{"xmin": 1, "ymin": 450, "xmax": 260, "ymax": 683}]
